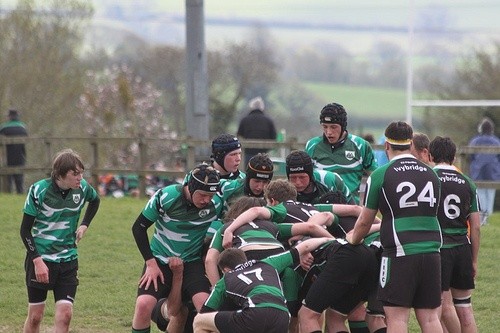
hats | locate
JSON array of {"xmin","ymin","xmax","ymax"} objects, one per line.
[
  {"xmin": 212, "ymin": 135, "xmax": 240, "ymax": 168},
  {"xmin": 320, "ymin": 103, "xmax": 347, "ymax": 140},
  {"xmin": 285, "ymin": 151, "xmax": 313, "ymax": 181},
  {"xmin": 9, "ymin": 111, "xmax": 18, "ymax": 119},
  {"xmin": 246, "ymin": 155, "xmax": 274, "ymax": 185},
  {"xmin": 189, "ymin": 165, "xmax": 220, "ymax": 198}
]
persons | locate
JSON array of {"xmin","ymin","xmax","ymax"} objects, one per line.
[
  {"xmin": 85, "ymin": 97, "xmax": 500, "ymax": 333},
  {"xmin": 19, "ymin": 150, "xmax": 101, "ymax": 333},
  {"xmin": 0, "ymin": 108, "xmax": 30, "ymax": 194}
]
